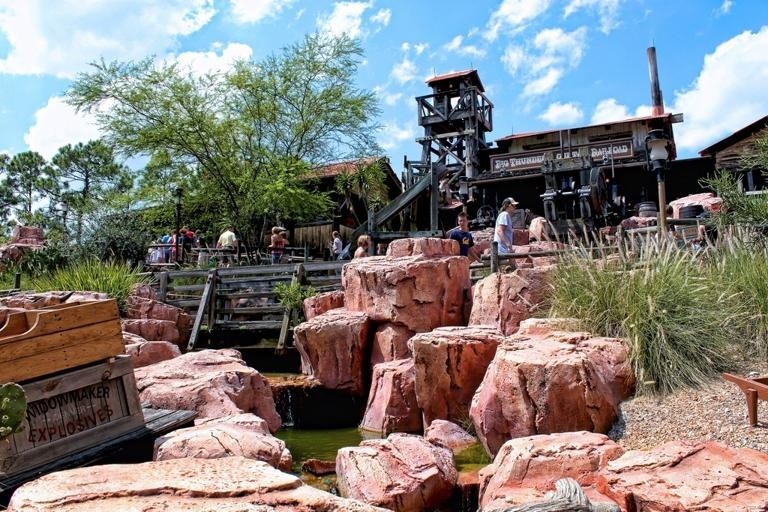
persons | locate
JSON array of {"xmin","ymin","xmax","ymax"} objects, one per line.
[
  {"xmin": 449, "ymin": 211, "xmax": 485, "ymax": 265},
  {"xmin": 278, "ymin": 231, "xmax": 290, "ymax": 247},
  {"xmin": 494, "ymin": 196, "xmax": 521, "ymax": 265},
  {"xmin": 267, "ymin": 226, "xmax": 284, "ymax": 264},
  {"xmin": 352, "ymin": 234, "xmax": 374, "ymax": 258},
  {"xmin": 215, "ymin": 224, "xmax": 238, "ymax": 255},
  {"xmin": 327, "ymin": 230, "xmax": 344, "ymax": 260},
  {"xmin": 694, "ymin": 197, "xmax": 726, "ymax": 247},
  {"xmin": 157, "ymin": 225, "xmax": 209, "ymax": 263}
]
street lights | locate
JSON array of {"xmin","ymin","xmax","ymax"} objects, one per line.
[
  {"xmin": 174, "ymin": 187, "xmax": 188, "ymax": 267},
  {"xmin": 642, "ymin": 128, "xmax": 673, "ymax": 232},
  {"xmin": 457, "ymin": 175, "xmax": 469, "ymax": 213}
]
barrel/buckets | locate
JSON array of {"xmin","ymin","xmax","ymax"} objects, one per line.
[
  {"xmin": 679, "ymin": 205, "xmax": 705, "ymax": 218},
  {"xmin": 639, "ymin": 201, "xmax": 657, "ymax": 217}
]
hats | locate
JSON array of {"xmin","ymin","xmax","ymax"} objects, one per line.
[{"xmin": 502, "ymin": 197, "xmax": 520, "ymax": 207}]
